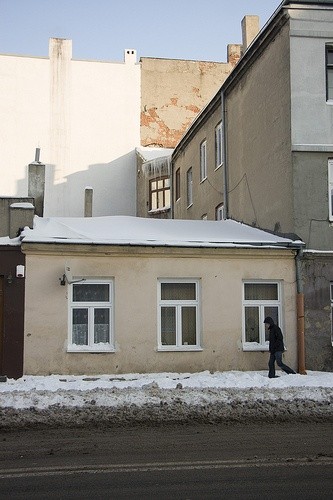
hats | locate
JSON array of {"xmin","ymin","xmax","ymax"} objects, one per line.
[{"xmin": 264, "ymin": 316, "xmax": 273, "ymax": 323}]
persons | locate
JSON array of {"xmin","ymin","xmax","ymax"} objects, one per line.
[{"xmin": 264, "ymin": 316, "xmax": 296, "ymax": 378}]
